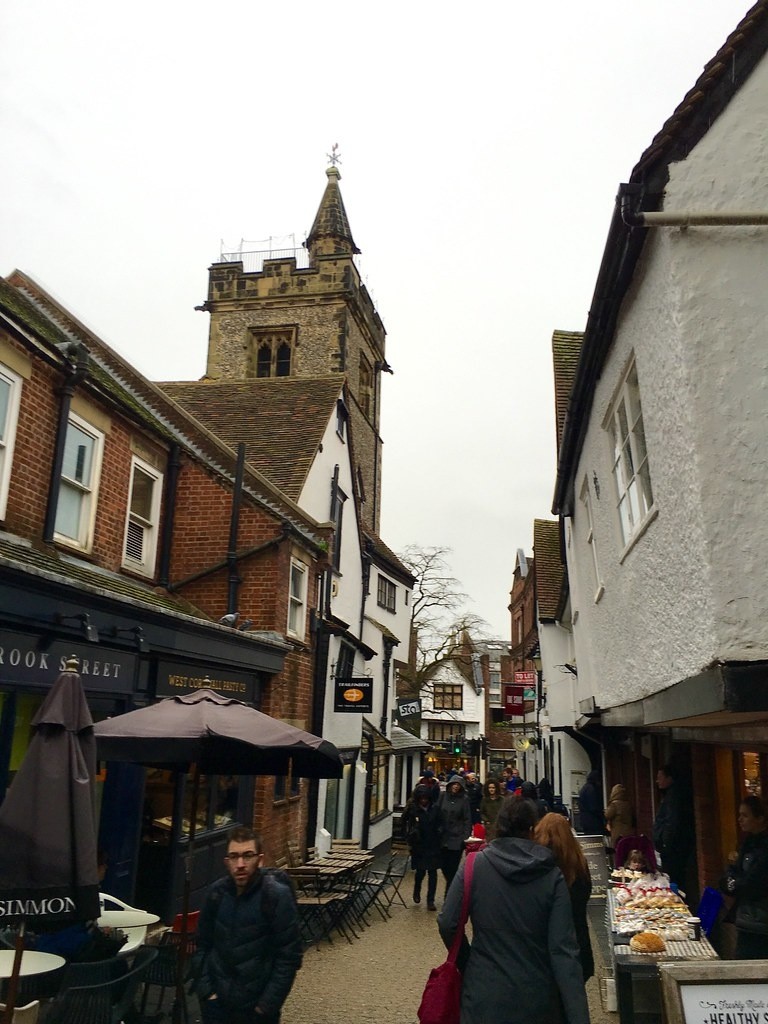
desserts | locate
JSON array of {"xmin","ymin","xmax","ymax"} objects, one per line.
[{"xmin": 612, "ymin": 869, "xmax": 691, "ymax": 941}]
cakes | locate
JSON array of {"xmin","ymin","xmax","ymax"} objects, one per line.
[{"xmin": 629, "ymin": 932, "xmax": 666, "ymax": 953}]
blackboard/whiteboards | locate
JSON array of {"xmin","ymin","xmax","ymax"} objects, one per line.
[{"xmin": 575, "ymin": 834, "xmax": 614, "ymax": 906}]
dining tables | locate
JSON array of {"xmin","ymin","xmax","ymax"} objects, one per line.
[{"xmin": 605, "ymin": 886, "xmax": 720, "ymax": 1023}]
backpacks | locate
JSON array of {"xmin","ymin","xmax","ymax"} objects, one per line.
[{"xmin": 207, "ymin": 867, "xmax": 302, "ymax": 970}]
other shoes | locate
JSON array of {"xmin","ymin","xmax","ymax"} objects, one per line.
[
  {"xmin": 413, "ymin": 890, "xmax": 421, "ymax": 903},
  {"xmin": 427, "ymin": 901, "xmax": 436, "ymax": 911}
]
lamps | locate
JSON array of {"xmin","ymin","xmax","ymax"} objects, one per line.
[
  {"xmin": 55, "ymin": 610, "xmax": 100, "ymax": 642},
  {"xmin": 113, "ymin": 624, "xmax": 151, "ymax": 653}
]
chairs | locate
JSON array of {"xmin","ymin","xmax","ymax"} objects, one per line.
[
  {"xmin": 43, "ymin": 946, "xmax": 159, "ymax": 1023},
  {"xmin": 93, "ymin": 892, "xmax": 149, "ymax": 953},
  {"xmin": 129, "ymin": 931, "xmax": 202, "ymax": 1024},
  {"xmin": 274, "ymin": 837, "xmax": 413, "ymax": 951},
  {"xmin": 0, "ymin": 931, "xmax": 51, "ymax": 998}
]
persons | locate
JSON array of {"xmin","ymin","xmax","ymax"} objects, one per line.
[
  {"xmin": 605, "ymin": 784, "xmax": 633, "ymax": 848},
  {"xmin": 578, "ymin": 769, "xmax": 603, "ymax": 835},
  {"xmin": 480, "ymin": 778, "xmax": 502, "ymax": 841},
  {"xmin": 534, "ymin": 812, "xmax": 595, "ymax": 985},
  {"xmin": 416, "ymin": 764, "xmax": 569, "ymax": 824},
  {"xmin": 458, "ymin": 823, "xmax": 487, "ymax": 865},
  {"xmin": 195, "ymin": 828, "xmax": 301, "ymax": 1024},
  {"xmin": 417, "ymin": 795, "xmax": 592, "ymax": 1024},
  {"xmin": 719, "ymin": 795, "xmax": 768, "ymax": 959},
  {"xmin": 434, "ymin": 775, "xmax": 471, "ymax": 905},
  {"xmin": 652, "ymin": 764, "xmax": 694, "ymax": 904},
  {"xmin": 401, "ymin": 784, "xmax": 445, "ymax": 911},
  {"xmin": 40, "ymin": 915, "xmax": 162, "ymax": 1024},
  {"xmin": 204, "ymin": 774, "xmax": 237, "ymax": 818},
  {"xmin": 623, "ymin": 850, "xmax": 654, "ymax": 874}
]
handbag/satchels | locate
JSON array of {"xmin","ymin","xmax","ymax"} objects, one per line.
[{"xmin": 416, "ymin": 963, "xmax": 461, "ymax": 1024}]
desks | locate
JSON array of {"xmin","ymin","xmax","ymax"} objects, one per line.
[
  {"xmin": 0, "ymin": 950, "xmax": 66, "ymax": 1023},
  {"xmin": 96, "ymin": 910, "xmax": 161, "ymax": 930}
]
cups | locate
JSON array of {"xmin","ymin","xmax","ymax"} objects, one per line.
[{"xmin": 686, "ymin": 917, "xmax": 701, "ymax": 941}]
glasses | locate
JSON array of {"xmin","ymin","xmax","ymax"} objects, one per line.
[{"xmin": 225, "ymin": 851, "xmax": 258, "ymax": 860}]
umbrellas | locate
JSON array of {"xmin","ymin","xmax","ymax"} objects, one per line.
[
  {"xmin": 92, "ymin": 673, "xmax": 345, "ymax": 1011},
  {"xmin": 0, "ymin": 652, "xmax": 102, "ymax": 1024}
]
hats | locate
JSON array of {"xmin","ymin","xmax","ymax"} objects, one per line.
[{"xmin": 473, "ymin": 823, "xmax": 486, "ymax": 839}]
